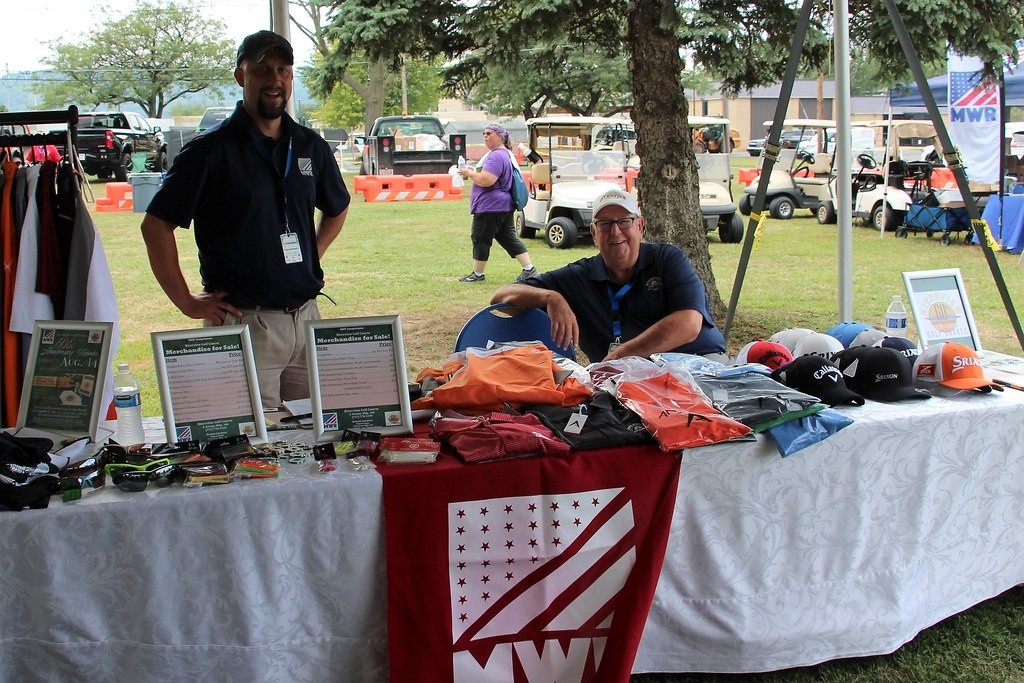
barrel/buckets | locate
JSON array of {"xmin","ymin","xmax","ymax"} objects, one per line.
[{"xmin": 127, "ymin": 172, "xmax": 162, "ymax": 213}]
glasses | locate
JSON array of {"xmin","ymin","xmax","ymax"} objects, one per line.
[
  {"xmin": 593, "ymin": 216, "xmax": 639, "ymax": 231},
  {"xmin": 54, "ymin": 442, "xmax": 179, "ymax": 496},
  {"xmin": 483, "ymin": 132, "xmax": 497, "ymax": 135}
]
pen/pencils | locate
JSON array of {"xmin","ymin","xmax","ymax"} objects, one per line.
[{"xmin": 992, "ymin": 379, "xmax": 1024, "ymax": 391}]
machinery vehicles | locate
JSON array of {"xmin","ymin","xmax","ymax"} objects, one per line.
[{"xmin": 691, "ymin": 98, "xmax": 742, "ymax": 154}]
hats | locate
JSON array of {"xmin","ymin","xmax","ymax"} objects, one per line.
[
  {"xmin": 593, "ymin": 189, "xmax": 638, "ymax": 215},
  {"xmin": 237, "ymin": 30, "xmax": 294, "ymax": 65},
  {"xmin": 734, "ymin": 322, "xmax": 1004, "ymax": 406}
]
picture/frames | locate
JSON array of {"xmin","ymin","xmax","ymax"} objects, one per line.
[{"xmin": 902, "ymin": 268, "xmax": 985, "ymax": 359}]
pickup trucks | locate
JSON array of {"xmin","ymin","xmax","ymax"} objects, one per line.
[
  {"xmin": 44, "ymin": 112, "xmax": 166, "ymax": 181},
  {"xmin": 354, "ymin": 116, "xmax": 467, "ymax": 176}
]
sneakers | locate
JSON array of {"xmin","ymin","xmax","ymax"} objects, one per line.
[
  {"xmin": 458, "ymin": 271, "xmax": 485, "ymax": 282},
  {"xmin": 515, "ymin": 266, "xmax": 538, "ymax": 283}
]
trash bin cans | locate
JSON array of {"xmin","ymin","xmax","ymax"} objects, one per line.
[{"xmin": 128, "ymin": 173, "xmax": 162, "ymax": 213}]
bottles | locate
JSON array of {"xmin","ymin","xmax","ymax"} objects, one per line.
[
  {"xmin": 458, "ymin": 155, "xmax": 466, "ymax": 169},
  {"xmin": 113, "ymin": 363, "xmax": 145, "ymax": 445},
  {"xmin": 885, "ymin": 295, "xmax": 907, "ymax": 339}
]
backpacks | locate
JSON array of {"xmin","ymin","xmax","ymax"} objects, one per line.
[{"xmin": 509, "ymin": 150, "xmax": 528, "ymax": 210}]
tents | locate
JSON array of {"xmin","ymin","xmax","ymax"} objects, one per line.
[{"xmin": 881, "ymin": 38, "xmax": 1024, "ymax": 236}]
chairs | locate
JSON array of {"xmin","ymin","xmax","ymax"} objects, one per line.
[
  {"xmin": 881, "ymin": 160, "xmax": 906, "ymax": 186},
  {"xmin": 797, "ymin": 153, "xmax": 830, "ymax": 184}
]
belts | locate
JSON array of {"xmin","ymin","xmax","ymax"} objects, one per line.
[{"xmin": 223, "ymin": 295, "xmax": 310, "ymax": 311}]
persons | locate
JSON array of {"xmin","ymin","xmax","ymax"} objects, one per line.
[
  {"xmin": 0, "ymin": 129, "xmax": 63, "ymax": 194},
  {"xmin": 490, "ymin": 190, "xmax": 730, "ymax": 364},
  {"xmin": 141, "ymin": 30, "xmax": 351, "ymax": 407},
  {"xmin": 458, "ymin": 123, "xmax": 538, "ymax": 285}
]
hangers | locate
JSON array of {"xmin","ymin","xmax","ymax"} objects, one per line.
[{"xmin": 0, "ymin": 129, "xmax": 95, "ymax": 203}]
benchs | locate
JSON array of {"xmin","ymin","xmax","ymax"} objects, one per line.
[{"xmin": 531, "ymin": 162, "xmax": 590, "ymax": 199}]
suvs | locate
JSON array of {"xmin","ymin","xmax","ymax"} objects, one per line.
[{"xmin": 194, "ymin": 107, "xmax": 235, "ymax": 137}]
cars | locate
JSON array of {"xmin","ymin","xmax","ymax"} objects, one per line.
[{"xmin": 747, "ymin": 131, "xmax": 816, "ymax": 156}]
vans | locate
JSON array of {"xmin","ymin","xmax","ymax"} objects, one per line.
[{"xmin": 798, "ymin": 128, "xmax": 876, "ymax": 152}]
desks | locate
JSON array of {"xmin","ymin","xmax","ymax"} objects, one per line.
[
  {"xmin": 1, "ymin": 346, "xmax": 1024, "ymax": 682},
  {"xmin": 942, "ymin": 187, "xmax": 1024, "ymax": 254}
]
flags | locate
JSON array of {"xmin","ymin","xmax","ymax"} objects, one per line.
[{"xmin": 946, "ymin": 36, "xmax": 1005, "ymax": 183}]
